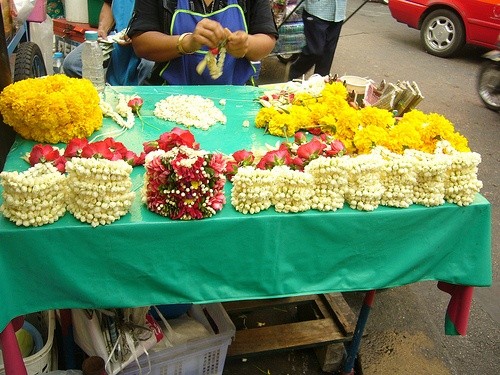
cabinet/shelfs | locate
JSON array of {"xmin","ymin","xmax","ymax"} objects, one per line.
[{"xmin": 52, "ymin": 18, "xmax": 99, "ymax": 59}]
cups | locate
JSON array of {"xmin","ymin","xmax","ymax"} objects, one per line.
[{"xmin": 339, "ymin": 75, "xmax": 371, "ymax": 108}]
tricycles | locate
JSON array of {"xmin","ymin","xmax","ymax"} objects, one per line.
[{"xmin": 0, "ymin": 1, "xmax": 48, "ymax": 85}]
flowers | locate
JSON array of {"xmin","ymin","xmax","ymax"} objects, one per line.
[
  {"xmin": 0, "ymin": 72, "xmax": 103, "ymax": 146},
  {"xmin": 195, "ymin": 29, "xmax": 231, "ymax": 80},
  {"xmin": 102, "ymin": 85, "xmax": 227, "ymax": 131},
  {"xmin": 66, "ymin": 156, "xmax": 134, "ymax": 227},
  {"xmin": 226, "ymin": 72, "xmax": 484, "ymax": 214},
  {"xmin": 143, "ymin": 144, "xmax": 225, "ymax": 221},
  {"xmin": 0, "ymin": 163, "xmax": 69, "ymax": 228},
  {"xmin": 21, "ymin": 122, "xmax": 201, "ymax": 166}
]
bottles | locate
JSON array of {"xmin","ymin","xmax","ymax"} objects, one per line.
[
  {"xmin": 82, "ymin": 30, "xmax": 105, "ymax": 104},
  {"xmin": 52, "ymin": 52, "xmax": 65, "ymax": 75}
]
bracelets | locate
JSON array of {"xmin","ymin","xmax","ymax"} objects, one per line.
[{"xmin": 177, "ymin": 32, "xmax": 196, "ymax": 56}]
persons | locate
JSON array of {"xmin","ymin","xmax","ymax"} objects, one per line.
[
  {"xmin": 127, "ymin": 0, "xmax": 279, "ymax": 86},
  {"xmin": 289, "ymin": 0, "xmax": 346, "ymax": 80},
  {"xmin": 63, "ymin": 0, "xmax": 145, "ymax": 87}
]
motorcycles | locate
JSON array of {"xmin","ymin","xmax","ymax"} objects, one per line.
[{"xmin": 475, "ymin": 49, "xmax": 500, "ymax": 112}]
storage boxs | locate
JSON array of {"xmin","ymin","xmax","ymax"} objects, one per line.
[
  {"xmin": 111, "ymin": 302, "xmax": 237, "ymax": 375},
  {"xmin": 26, "ymin": 0, "xmax": 47, "ymax": 24},
  {"xmin": 0, "ymin": 309, "xmax": 56, "ymax": 375}
]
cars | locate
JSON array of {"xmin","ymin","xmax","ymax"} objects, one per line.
[{"xmin": 387, "ymin": 0, "xmax": 500, "ymax": 57}]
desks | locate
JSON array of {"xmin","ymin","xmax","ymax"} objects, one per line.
[{"xmin": 0, "ymin": 84, "xmax": 494, "ymax": 336}]
